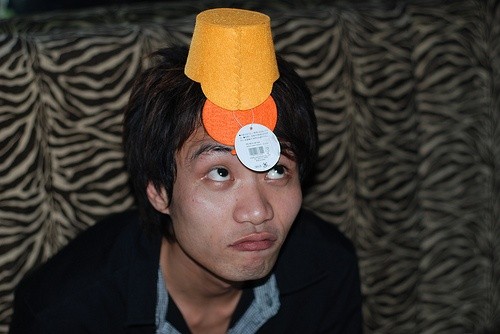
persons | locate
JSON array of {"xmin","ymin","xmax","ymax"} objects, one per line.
[{"xmin": 10, "ymin": 44, "xmax": 365, "ymax": 334}]
